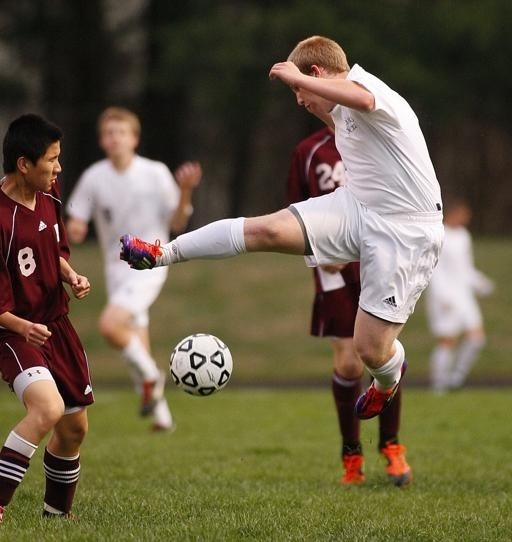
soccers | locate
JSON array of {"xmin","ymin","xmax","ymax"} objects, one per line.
[{"xmin": 169, "ymin": 334, "xmax": 233, "ymax": 397}]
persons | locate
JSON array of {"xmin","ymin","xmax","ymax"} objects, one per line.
[
  {"xmin": 63, "ymin": 106, "xmax": 204, "ymax": 433},
  {"xmin": 422, "ymin": 195, "xmax": 496, "ymax": 398},
  {"xmin": 118, "ymin": 33, "xmax": 449, "ymax": 420},
  {"xmin": 0, "ymin": 112, "xmax": 94, "ymax": 522},
  {"xmin": 281, "ymin": 124, "xmax": 415, "ymax": 489}
]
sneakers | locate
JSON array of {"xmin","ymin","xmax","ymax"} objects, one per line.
[
  {"xmin": 118, "ymin": 234, "xmax": 161, "ymax": 269},
  {"xmin": 342, "ymin": 453, "xmax": 364, "ymax": 487},
  {"xmin": 355, "ymin": 364, "xmax": 407, "ymax": 420},
  {"xmin": 376, "ymin": 439, "xmax": 412, "ymax": 487},
  {"xmin": 151, "ymin": 418, "xmax": 179, "ymax": 435},
  {"xmin": 136, "ymin": 370, "xmax": 167, "ymax": 420}
]
